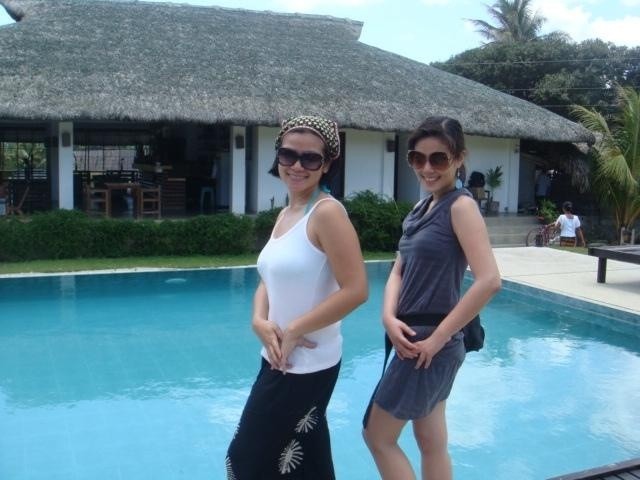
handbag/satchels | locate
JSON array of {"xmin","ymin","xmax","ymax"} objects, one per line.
[{"xmin": 463, "ymin": 315, "xmax": 484, "ymax": 352}]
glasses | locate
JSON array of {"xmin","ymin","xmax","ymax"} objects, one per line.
[
  {"xmin": 278, "ymin": 148, "xmax": 323, "ymax": 170},
  {"xmin": 407, "ymin": 150, "xmax": 449, "ymax": 169}
]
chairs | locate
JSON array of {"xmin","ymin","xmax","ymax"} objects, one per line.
[{"xmin": 85, "ymin": 175, "xmax": 162, "ymax": 221}]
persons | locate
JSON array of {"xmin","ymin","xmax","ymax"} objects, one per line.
[
  {"xmin": 223, "ymin": 113, "xmax": 372, "ymax": 479},
  {"xmin": 553, "ymin": 200, "xmax": 586, "ymax": 247},
  {"xmin": 360, "ymin": 114, "xmax": 502, "ymax": 480},
  {"xmin": 534, "ymin": 169, "xmax": 552, "ymax": 209}
]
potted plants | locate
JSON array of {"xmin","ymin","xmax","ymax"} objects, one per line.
[{"xmin": 485, "ymin": 166, "xmax": 502, "ymax": 215}]
